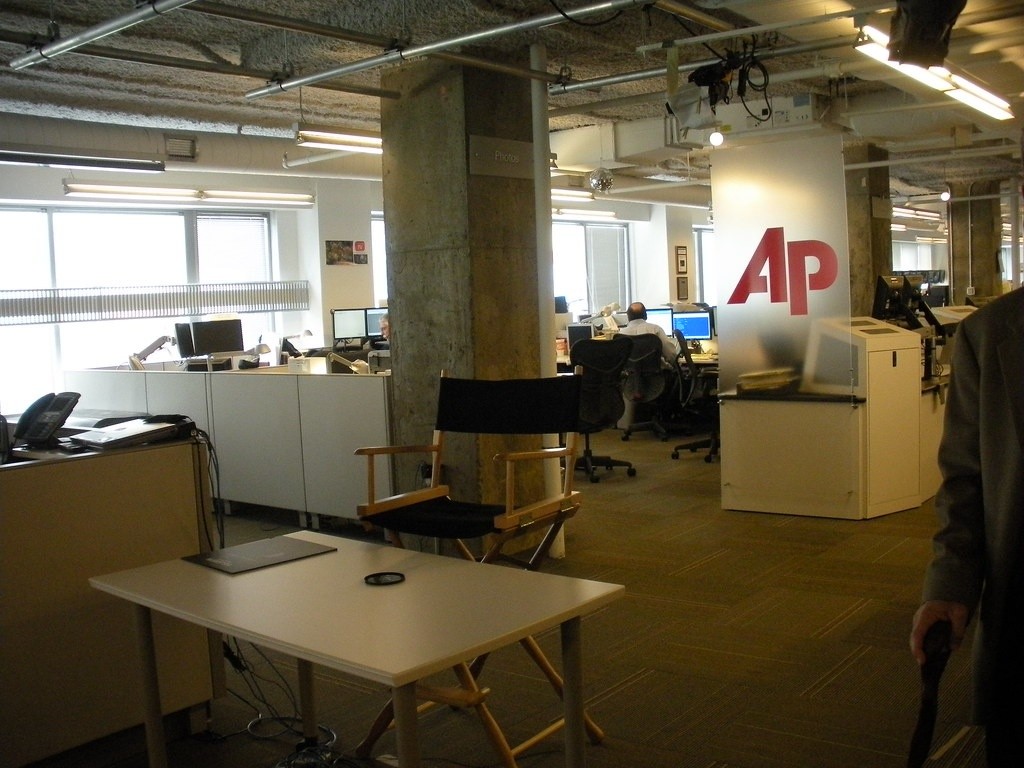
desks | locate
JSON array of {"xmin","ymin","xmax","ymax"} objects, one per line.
[
  {"xmin": 87, "ymin": 529, "xmax": 625, "ymax": 768},
  {"xmin": 679, "ymin": 349, "xmax": 716, "ymax": 371}
]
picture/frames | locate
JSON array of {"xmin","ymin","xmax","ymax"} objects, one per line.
[
  {"xmin": 675, "ymin": 246, "xmax": 688, "ymax": 273},
  {"xmin": 677, "ymin": 277, "xmax": 688, "ymax": 300}
]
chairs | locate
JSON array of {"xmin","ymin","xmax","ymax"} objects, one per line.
[{"xmin": 555, "ymin": 332, "xmax": 721, "ymax": 482}]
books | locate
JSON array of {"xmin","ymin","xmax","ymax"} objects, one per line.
[{"xmin": 69, "ymin": 418, "xmax": 196, "ymax": 451}]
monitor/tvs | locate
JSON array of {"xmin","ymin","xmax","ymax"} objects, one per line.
[
  {"xmin": 136, "ymin": 319, "xmax": 244, "ymax": 363},
  {"xmin": 872, "ymin": 274, "xmax": 923, "ymax": 321},
  {"xmin": 567, "ymin": 323, "xmax": 595, "ymax": 352},
  {"xmin": 331, "ymin": 307, "xmax": 389, "ymax": 350},
  {"xmin": 644, "ymin": 308, "xmax": 712, "ymax": 341}
]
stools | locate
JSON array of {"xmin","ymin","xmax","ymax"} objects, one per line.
[{"xmin": 351, "ymin": 372, "xmax": 603, "ymax": 768}]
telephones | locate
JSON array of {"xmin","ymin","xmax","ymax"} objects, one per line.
[{"xmin": 13, "ymin": 392, "xmax": 81, "ymax": 441}]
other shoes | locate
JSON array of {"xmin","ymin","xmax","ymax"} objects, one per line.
[{"xmin": 669, "ymin": 412, "xmax": 683, "ymax": 421}]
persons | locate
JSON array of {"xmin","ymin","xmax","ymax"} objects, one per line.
[
  {"xmin": 379, "ymin": 313, "xmax": 389, "ymax": 341},
  {"xmin": 616, "ymin": 302, "xmax": 677, "ymax": 430},
  {"xmin": 911, "ymin": 286, "xmax": 1024, "ymax": 768}
]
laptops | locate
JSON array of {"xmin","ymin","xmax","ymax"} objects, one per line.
[{"xmin": 70, "ymin": 417, "xmax": 178, "ymax": 453}]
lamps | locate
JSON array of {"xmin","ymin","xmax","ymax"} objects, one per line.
[
  {"xmin": 280, "ymin": 330, "xmax": 311, "ymax": 357},
  {"xmin": 710, "ymin": 120, "xmax": 725, "ymax": 147},
  {"xmin": 940, "ymin": 161, "xmax": 950, "ymax": 202},
  {"xmin": 66, "ymin": 178, "xmax": 316, "ymax": 207},
  {"xmin": 589, "ymin": 124, "xmax": 615, "ymax": 192},
  {"xmin": 328, "ymin": 352, "xmax": 369, "ymax": 376},
  {"xmin": 207, "ymin": 343, "xmax": 272, "ymax": 372},
  {"xmin": 291, "ymin": 122, "xmax": 559, "ymax": 171},
  {"xmin": 845, "ymin": 26, "xmax": 1015, "ymax": 122},
  {"xmin": 580, "ymin": 302, "xmax": 620, "ymax": 323},
  {"xmin": 892, "ymin": 205, "xmax": 1023, "ymax": 245}
]
